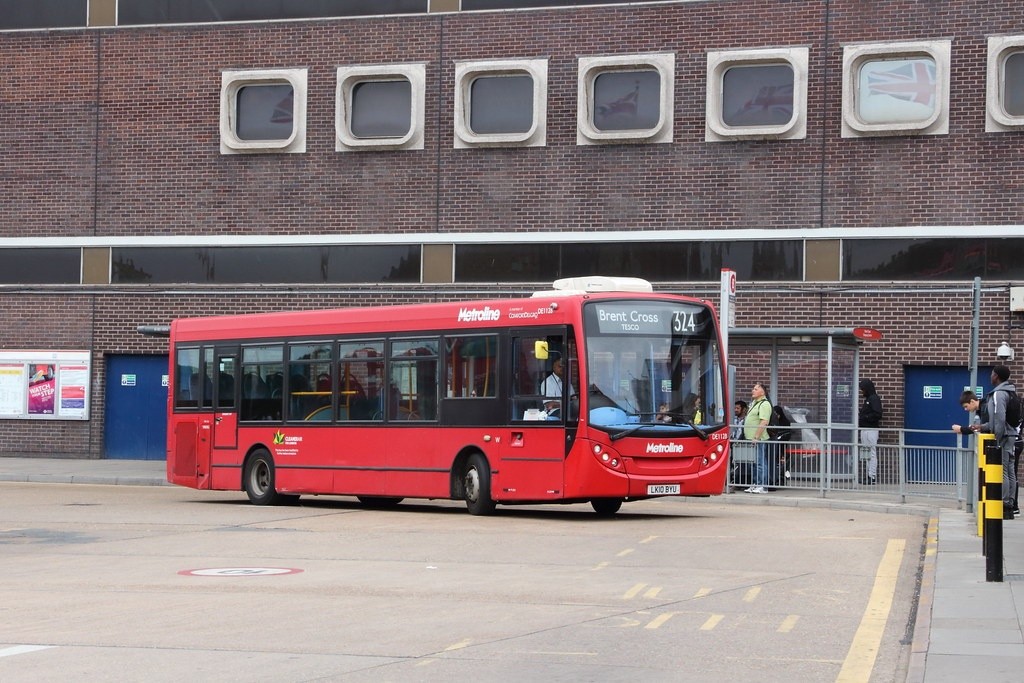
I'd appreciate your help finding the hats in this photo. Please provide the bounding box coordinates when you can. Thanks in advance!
[994,365,1010,379]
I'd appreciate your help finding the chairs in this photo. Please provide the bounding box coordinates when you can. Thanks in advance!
[189,372,365,419]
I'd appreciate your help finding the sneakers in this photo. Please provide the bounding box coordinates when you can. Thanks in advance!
[744,484,756,492]
[752,487,768,493]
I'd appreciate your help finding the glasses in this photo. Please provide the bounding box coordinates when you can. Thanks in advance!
[470,394,475,397]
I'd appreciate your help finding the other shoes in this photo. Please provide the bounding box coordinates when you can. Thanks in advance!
[769,488,776,490]
[1013,508,1020,514]
[785,471,790,478]
[859,476,875,484]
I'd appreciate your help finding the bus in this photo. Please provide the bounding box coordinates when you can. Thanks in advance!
[136,276,731,516]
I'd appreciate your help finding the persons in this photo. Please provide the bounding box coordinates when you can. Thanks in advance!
[656,382,791,494]
[952,365,1024,520]
[859,378,882,485]
[541,360,577,419]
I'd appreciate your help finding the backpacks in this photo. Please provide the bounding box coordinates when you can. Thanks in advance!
[992,389,1020,427]
[758,400,780,436]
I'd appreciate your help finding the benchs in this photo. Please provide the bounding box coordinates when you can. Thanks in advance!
[786,449,858,488]
